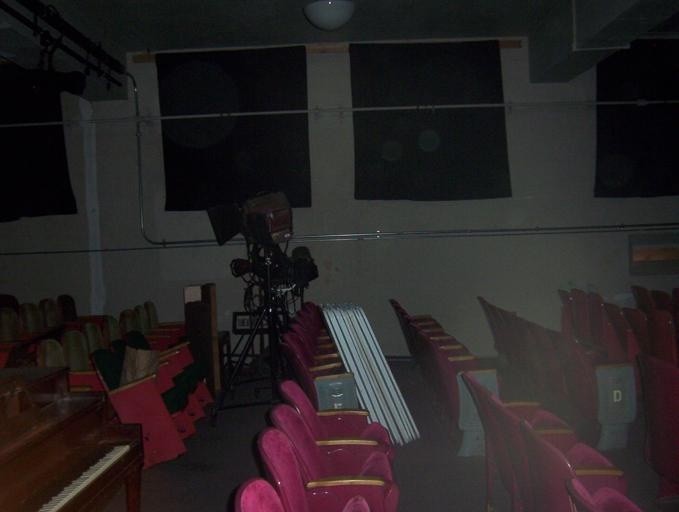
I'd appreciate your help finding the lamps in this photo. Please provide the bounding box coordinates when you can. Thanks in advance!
[301,0,356,32]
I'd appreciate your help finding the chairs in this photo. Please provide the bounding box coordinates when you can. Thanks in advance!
[461,371,541,510]
[259,427,400,511]
[478,385,578,508]
[636,351,678,503]
[233,474,370,511]
[564,476,640,509]
[268,404,395,463]
[2,292,216,472]
[277,380,394,447]
[281,286,677,440]
[522,418,628,509]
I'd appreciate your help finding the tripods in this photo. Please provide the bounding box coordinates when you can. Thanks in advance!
[208,249,280,416]
